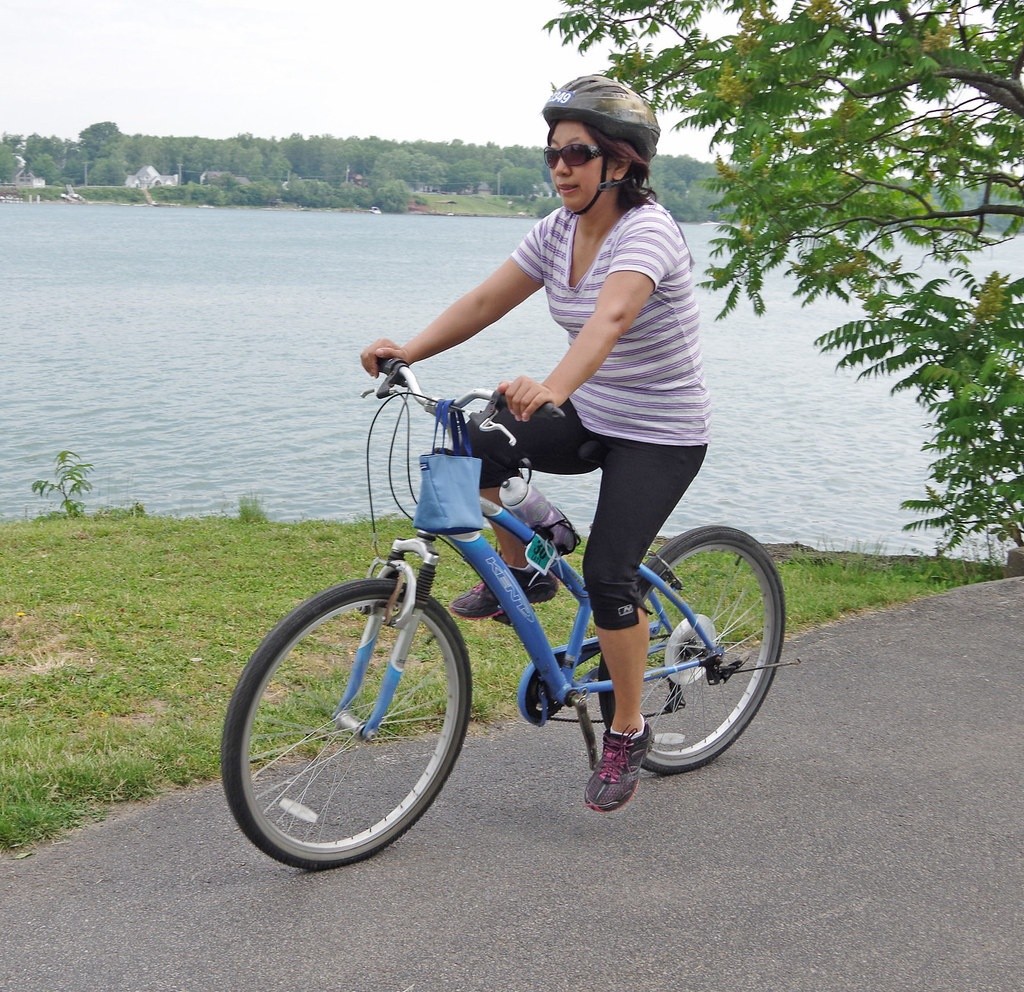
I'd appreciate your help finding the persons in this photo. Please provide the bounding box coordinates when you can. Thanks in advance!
[360,76,712,813]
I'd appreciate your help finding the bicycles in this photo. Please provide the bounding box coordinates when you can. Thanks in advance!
[218,350,790,872]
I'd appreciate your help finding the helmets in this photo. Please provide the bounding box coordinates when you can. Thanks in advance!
[542,75,662,161]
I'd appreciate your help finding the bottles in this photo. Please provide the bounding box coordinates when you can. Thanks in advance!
[499,477,578,550]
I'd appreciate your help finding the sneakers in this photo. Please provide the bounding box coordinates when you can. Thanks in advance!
[584,711,655,811]
[449,550,558,621]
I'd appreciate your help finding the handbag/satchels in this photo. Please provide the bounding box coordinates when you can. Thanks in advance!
[413,398,484,535]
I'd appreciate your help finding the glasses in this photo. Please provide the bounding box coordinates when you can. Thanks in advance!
[543,143,604,170]
